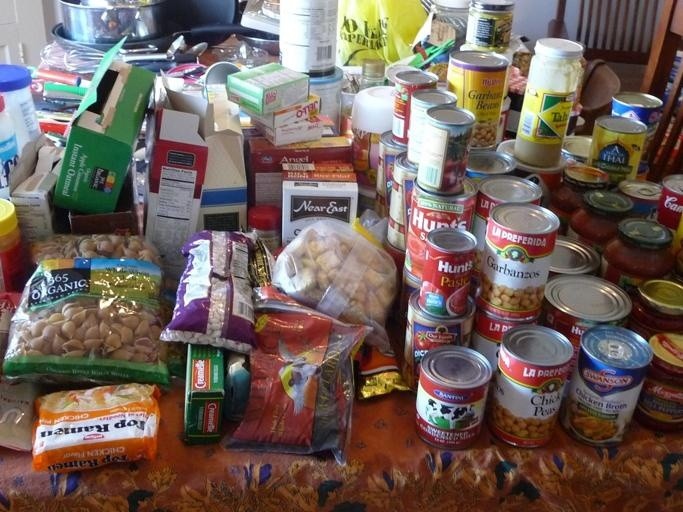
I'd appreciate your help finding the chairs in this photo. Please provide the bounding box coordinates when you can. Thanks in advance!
[639,0,683,183]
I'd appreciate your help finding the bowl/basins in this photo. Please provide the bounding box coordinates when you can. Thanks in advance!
[57,0,179,41]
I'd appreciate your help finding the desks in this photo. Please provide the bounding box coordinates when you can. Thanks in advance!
[0,319,682,512]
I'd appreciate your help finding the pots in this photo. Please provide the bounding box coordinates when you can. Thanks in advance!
[46,20,270,53]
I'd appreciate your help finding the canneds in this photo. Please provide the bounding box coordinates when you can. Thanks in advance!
[391,69,439,147]
[376,129,479,290]
[415,346,492,450]
[418,227,478,319]
[279,0,343,137]
[611,92,664,142]
[560,136,592,165]
[407,90,457,171]
[584,115,648,183]
[401,288,477,392]
[445,50,508,150]
[464,139,576,280]
[469,308,541,383]
[471,202,560,322]
[559,325,653,448]
[538,274,633,400]
[486,325,574,448]
[417,105,476,197]
[546,165,683,434]
